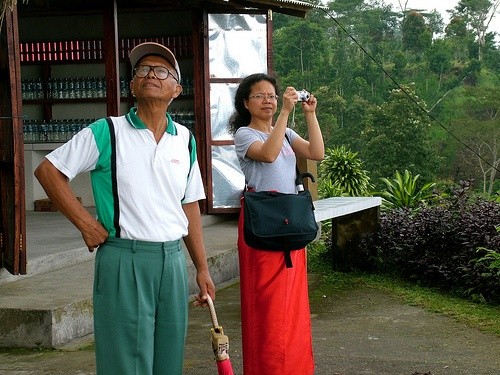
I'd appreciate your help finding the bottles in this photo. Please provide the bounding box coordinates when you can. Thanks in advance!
[121,77,134,97]
[168,108,194,130]
[21,77,106,98]
[181,72,193,93]
[22,118,93,142]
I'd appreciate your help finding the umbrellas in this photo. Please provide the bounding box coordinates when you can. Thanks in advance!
[205,294,234,375]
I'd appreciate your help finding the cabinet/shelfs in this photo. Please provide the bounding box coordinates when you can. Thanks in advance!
[117,37,195,133]
[20,41,48,142]
[47,41,112,143]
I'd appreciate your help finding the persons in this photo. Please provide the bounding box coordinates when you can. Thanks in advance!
[34,43,216,375]
[228,73,324,375]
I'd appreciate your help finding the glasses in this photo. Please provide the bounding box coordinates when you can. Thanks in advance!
[247,94,279,101]
[132,65,179,84]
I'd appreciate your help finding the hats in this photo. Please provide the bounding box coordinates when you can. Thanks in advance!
[128,42,181,105]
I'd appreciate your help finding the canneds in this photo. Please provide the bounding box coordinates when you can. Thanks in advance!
[19,40,103,62]
[119,34,192,59]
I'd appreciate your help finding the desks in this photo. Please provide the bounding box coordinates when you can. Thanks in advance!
[313,196,382,273]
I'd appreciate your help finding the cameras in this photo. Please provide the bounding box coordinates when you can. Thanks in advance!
[294,91,310,101]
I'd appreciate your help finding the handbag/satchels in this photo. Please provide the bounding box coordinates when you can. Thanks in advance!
[243,190,319,251]
